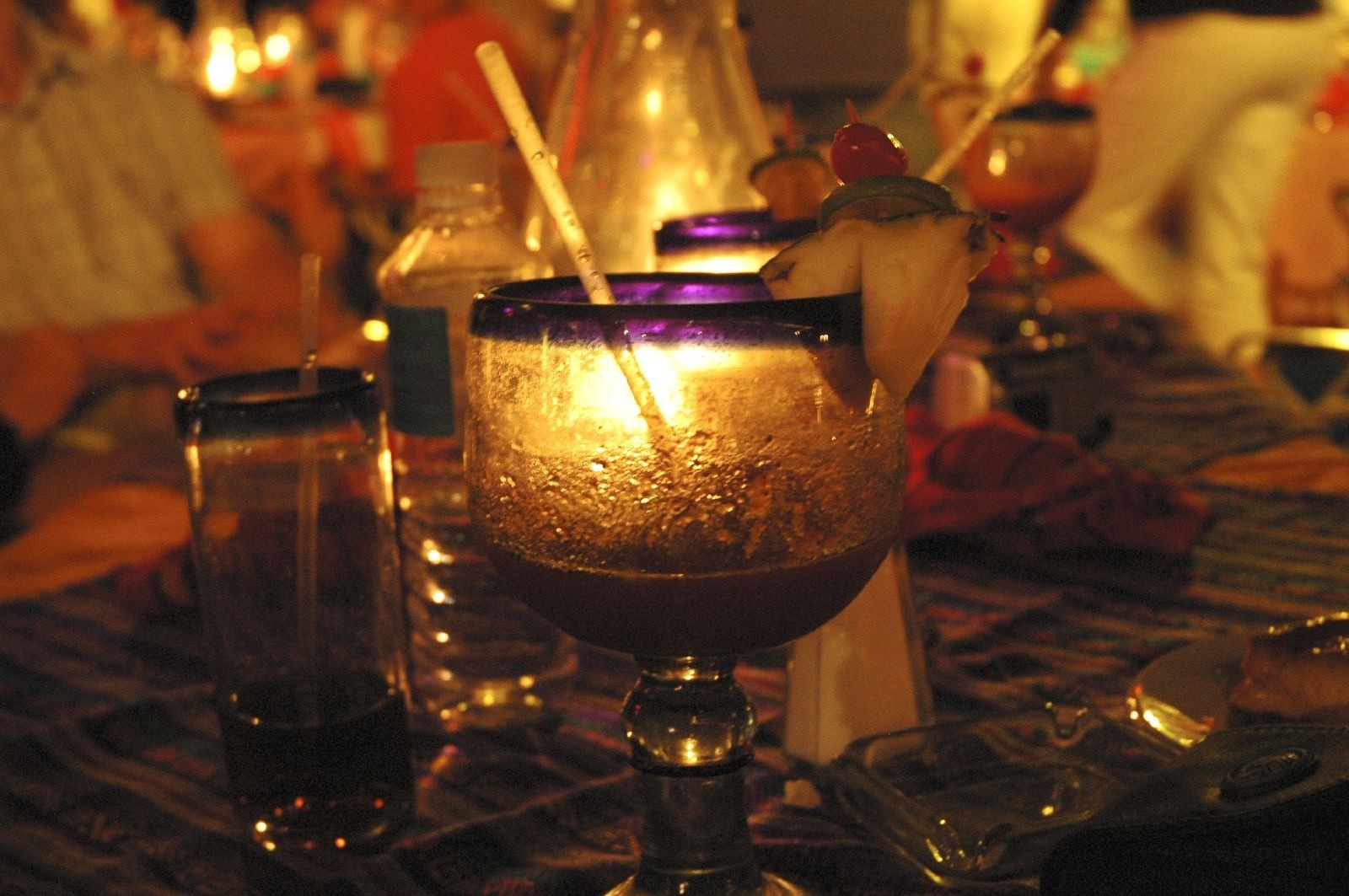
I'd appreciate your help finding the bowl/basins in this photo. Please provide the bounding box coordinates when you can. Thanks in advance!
[961,305,1163,449]
[1266,326,1349,444]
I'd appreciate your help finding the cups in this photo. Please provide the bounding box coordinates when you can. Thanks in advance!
[657,208,822,274]
[173,361,417,853]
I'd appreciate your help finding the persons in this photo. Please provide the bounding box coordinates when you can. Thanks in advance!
[317,0,553,277]
[0,0,306,528]
[0,414,43,542]
[1071,1,1349,425]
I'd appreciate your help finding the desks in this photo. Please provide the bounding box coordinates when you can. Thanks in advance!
[0,306,1349,896]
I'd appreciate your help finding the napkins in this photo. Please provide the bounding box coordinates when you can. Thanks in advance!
[907,414,1218,558]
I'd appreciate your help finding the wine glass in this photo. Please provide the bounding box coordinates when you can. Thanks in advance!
[459,274,907,896]
[954,95,1102,313]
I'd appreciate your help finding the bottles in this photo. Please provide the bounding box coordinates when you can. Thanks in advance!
[524,0,799,273]
[371,139,578,729]
